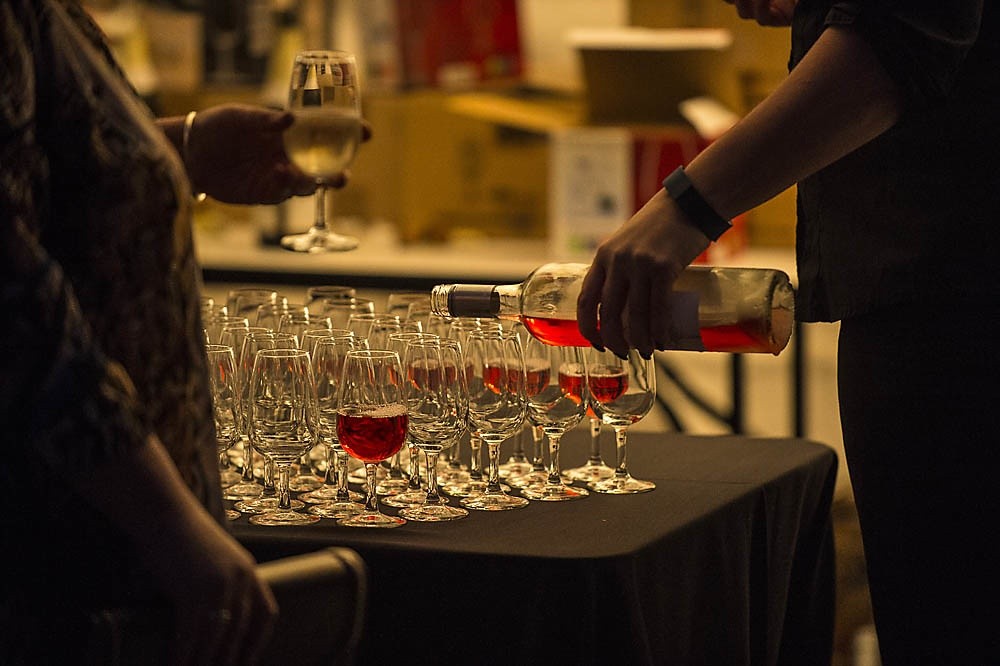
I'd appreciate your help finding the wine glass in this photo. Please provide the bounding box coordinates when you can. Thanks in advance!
[218,327,322,526]
[366,320,660,522]
[202,344,241,522]
[300,330,407,528]
[279,50,361,254]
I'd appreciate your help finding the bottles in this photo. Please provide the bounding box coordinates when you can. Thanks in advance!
[430,261,796,354]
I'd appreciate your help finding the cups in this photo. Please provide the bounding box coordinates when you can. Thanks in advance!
[202,285,500,327]
[216,316,249,389]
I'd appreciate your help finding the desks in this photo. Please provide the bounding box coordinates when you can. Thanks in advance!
[437,89,749,269]
[227,430,838,666]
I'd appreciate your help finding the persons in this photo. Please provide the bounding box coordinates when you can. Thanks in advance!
[0,0,375,666]
[576,0,1000,666]
[178,110,206,203]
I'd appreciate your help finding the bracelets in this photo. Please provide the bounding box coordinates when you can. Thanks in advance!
[662,165,733,242]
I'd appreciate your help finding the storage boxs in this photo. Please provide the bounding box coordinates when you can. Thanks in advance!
[569,25,735,121]
